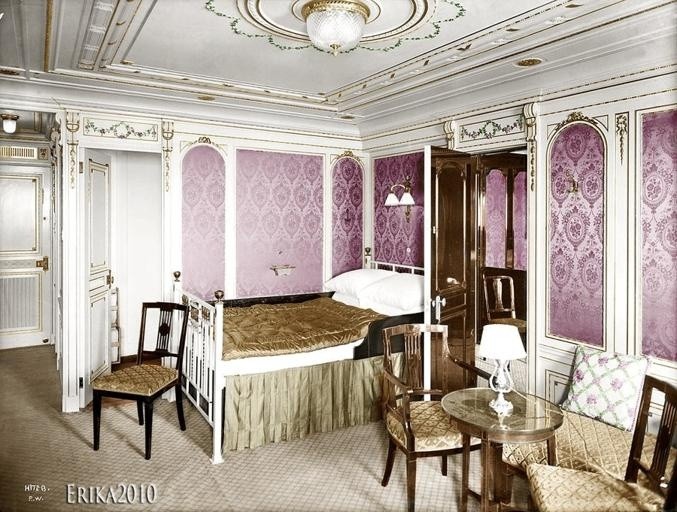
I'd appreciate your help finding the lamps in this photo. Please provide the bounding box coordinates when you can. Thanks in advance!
[301,0,370,56]
[478,324,528,407]
[2,114,19,135]
[384,182,415,206]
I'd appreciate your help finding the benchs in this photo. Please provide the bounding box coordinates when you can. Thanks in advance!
[499,410,677,491]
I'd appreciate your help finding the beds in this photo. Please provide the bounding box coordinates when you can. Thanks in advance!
[168,247,425,465]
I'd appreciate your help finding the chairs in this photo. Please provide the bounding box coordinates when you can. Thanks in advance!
[378,324,481,512]
[525,373,677,512]
[91,302,189,460]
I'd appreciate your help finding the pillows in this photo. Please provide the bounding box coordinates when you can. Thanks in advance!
[560,344,648,431]
[325,268,424,317]
